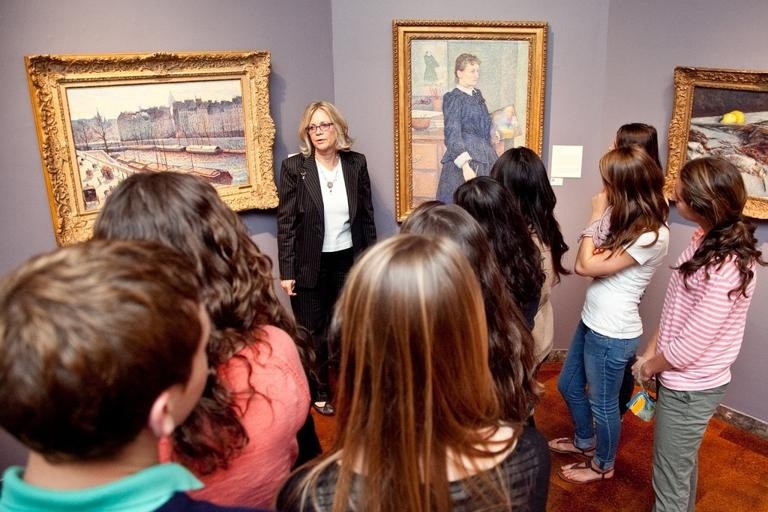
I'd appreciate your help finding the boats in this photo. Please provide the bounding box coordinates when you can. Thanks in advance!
[88,144,246,182]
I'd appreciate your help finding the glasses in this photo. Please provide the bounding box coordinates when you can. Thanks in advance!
[305,121,332,135]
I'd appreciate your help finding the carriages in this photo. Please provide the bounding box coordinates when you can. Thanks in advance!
[82,182,100,208]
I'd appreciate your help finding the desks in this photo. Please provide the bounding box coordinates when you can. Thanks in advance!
[411,119,514,198]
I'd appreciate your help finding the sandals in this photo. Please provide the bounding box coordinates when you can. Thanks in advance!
[548,436,596,457]
[558,457,614,483]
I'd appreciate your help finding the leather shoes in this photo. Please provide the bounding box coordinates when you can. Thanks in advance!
[314,402,334,414]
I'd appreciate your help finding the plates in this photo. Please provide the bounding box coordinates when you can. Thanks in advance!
[432,120,444,128]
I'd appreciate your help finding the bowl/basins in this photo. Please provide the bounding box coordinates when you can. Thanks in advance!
[412,118,430,129]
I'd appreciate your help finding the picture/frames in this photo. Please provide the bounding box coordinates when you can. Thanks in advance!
[662,65,768,221]
[23,48,280,249]
[390,17,547,224]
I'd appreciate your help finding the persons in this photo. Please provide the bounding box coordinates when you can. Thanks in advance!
[275,231,550,511]
[611,122,664,428]
[423,49,440,82]
[0,237,270,511]
[547,147,671,484]
[490,105,524,154]
[496,145,573,379]
[276,101,378,415]
[397,200,535,429]
[626,154,768,512]
[435,53,498,203]
[455,175,547,331]
[90,168,326,510]
